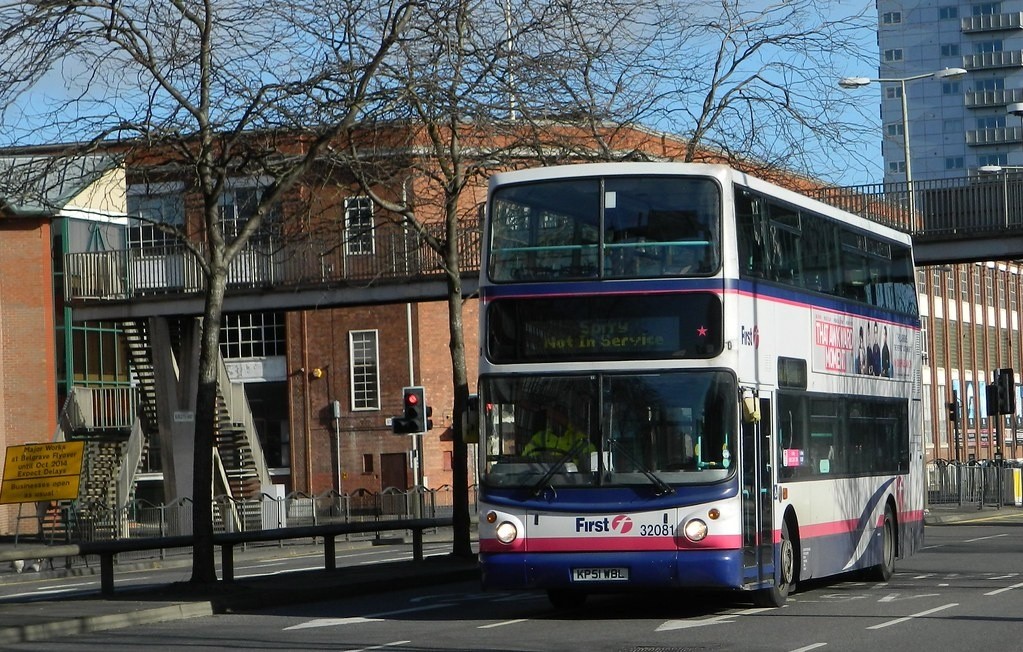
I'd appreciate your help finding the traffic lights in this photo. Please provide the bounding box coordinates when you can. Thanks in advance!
[425,405,433,430]
[391,387,427,435]
[949,403,956,422]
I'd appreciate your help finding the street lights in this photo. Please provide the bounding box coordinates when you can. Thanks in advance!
[839,67,968,234]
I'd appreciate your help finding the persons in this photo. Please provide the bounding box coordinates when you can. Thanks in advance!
[858,320,890,377]
[521,404,597,473]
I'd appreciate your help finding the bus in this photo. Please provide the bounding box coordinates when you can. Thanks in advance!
[462,161,926,607]
